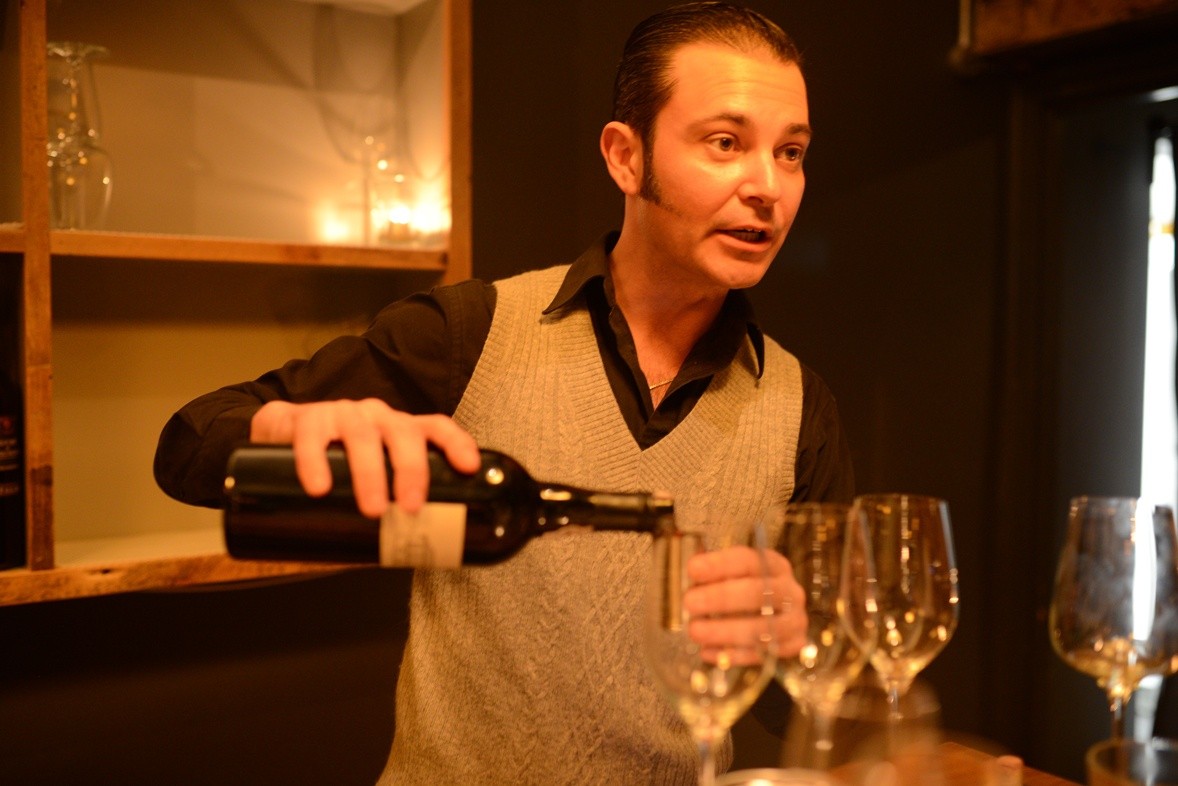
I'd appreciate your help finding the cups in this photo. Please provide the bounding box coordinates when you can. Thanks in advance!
[1085,736,1178,786]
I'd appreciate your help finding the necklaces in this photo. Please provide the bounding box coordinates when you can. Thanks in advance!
[649,377,676,391]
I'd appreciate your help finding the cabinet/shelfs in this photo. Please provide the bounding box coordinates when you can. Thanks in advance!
[0,1,472,605]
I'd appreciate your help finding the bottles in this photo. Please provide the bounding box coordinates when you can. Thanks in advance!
[222,443,677,569]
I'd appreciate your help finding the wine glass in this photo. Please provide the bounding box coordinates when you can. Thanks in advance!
[640,509,780,786]
[753,501,878,780]
[46,41,114,231]
[1047,494,1178,739]
[839,491,958,761]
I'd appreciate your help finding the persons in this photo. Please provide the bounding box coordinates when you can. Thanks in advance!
[152,9,859,786]
